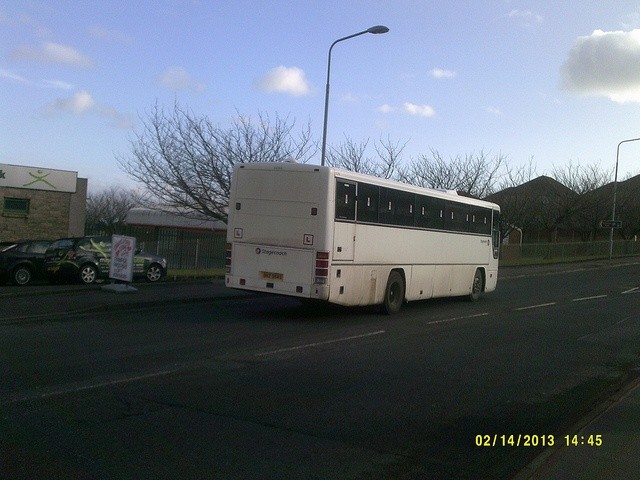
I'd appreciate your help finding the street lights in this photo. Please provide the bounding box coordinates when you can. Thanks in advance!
[596,136,639,262]
[320,25,390,167]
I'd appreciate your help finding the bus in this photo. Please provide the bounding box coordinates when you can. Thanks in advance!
[224,162,501,316]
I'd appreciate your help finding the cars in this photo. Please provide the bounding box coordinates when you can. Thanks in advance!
[0,239,51,286]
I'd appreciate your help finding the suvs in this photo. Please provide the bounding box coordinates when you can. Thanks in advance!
[38,236,168,286]
